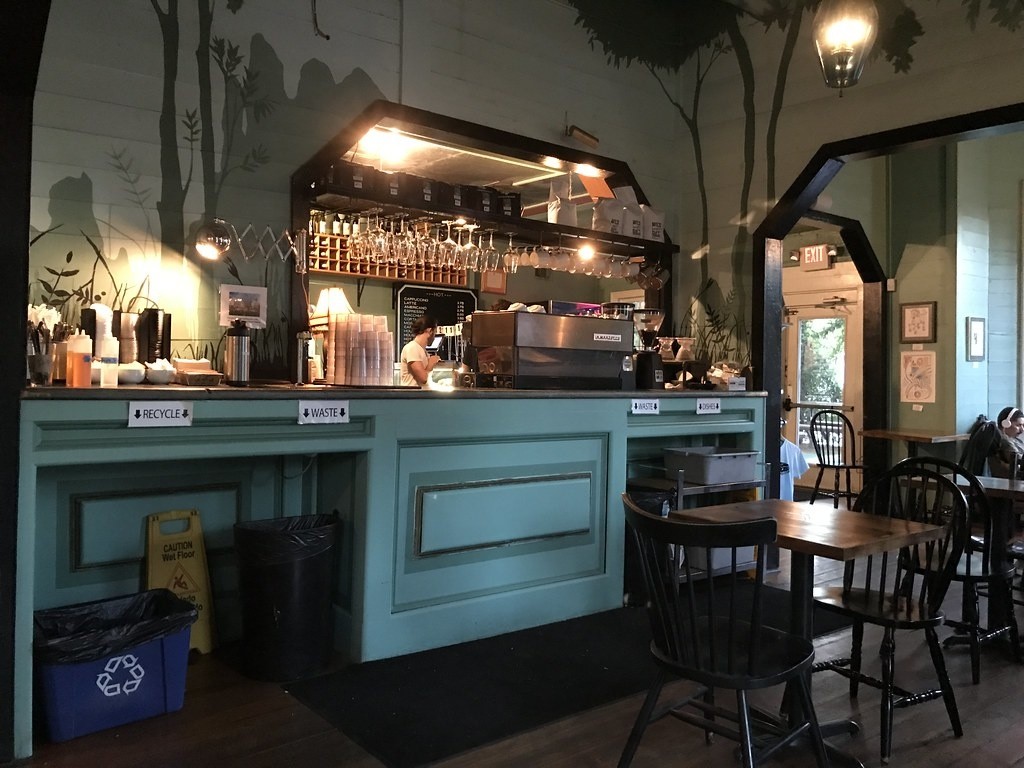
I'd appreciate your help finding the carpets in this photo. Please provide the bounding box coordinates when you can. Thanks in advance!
[280,574,865,768]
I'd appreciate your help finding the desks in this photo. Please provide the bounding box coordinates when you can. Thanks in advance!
[858,429,972,520]
[896,474,1024,663]
[667,500,947,767]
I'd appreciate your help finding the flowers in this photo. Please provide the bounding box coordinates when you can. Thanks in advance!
[27,302,60,354]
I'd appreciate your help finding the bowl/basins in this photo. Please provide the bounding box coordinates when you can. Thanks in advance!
[91,369,101,382]
[118,369,145,386]
[145,369,176,384]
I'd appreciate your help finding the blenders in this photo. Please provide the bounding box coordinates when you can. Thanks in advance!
[632,308,667,390]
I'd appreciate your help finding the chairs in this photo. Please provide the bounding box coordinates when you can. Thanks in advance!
[811,469,971,767]
[811,409,869,511]
[617,490,833,767]
[898,417,1024,645]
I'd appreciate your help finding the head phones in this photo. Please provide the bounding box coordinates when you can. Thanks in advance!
[1002,408,1019,428]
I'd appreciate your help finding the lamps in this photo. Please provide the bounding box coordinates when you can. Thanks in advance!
[195,220,233,263]
[567,125,598,149]
[813,0,880,98]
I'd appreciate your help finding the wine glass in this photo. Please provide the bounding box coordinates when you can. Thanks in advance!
[348,207,520,274]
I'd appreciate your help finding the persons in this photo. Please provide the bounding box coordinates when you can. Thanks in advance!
[460,300,514,388]
[988,407,1024,483]
[400,314,441,389]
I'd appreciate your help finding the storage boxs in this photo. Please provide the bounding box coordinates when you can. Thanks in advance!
[710,375,747,392]
[686,545,754,571]
[662,445,762,484]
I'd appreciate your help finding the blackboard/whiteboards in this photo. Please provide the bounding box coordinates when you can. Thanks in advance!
[394,282,479,363]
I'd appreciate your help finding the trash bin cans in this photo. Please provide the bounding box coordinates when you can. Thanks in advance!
[33,588,199,747]
[233,513,340,682]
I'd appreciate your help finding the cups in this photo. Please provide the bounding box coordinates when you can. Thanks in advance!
[328,313,394,386]
[505,246,670,291]
[27,354,56,388]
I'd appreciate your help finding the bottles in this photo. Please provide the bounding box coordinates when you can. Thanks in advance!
[66,327,79,386]
[101,331,119,388]
[225,318,250,386]
[308,210,449,277]
[73,330,92,387]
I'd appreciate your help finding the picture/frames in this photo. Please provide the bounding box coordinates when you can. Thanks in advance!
[899,300,938,344]
[219,283,269,331]
[965,317,986,363]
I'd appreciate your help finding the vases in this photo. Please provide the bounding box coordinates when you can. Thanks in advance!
[28,353,56,388]
